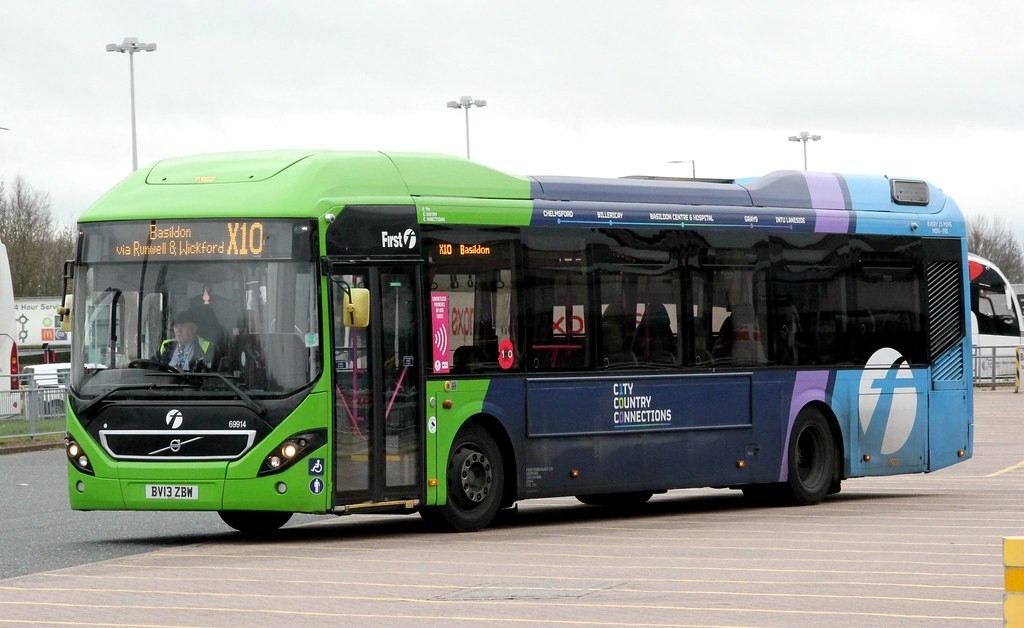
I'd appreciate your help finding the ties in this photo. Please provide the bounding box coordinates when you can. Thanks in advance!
[175,345,185,368]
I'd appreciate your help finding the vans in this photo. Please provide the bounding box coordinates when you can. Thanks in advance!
[21,362,108,412]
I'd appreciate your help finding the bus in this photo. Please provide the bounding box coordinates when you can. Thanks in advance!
[56,172,973,531]
[966,251,1024,387]
[0,244,22,418]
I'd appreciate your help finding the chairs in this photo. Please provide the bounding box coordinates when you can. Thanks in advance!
[160,301,922,414]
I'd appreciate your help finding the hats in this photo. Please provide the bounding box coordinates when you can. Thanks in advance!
[173,310,197,325]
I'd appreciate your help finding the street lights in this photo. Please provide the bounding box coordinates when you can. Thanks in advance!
[107,38,157,170]
[448,96,486,159]
[789,132,820,171]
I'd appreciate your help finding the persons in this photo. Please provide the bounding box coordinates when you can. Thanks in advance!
[142,311,219,391]
